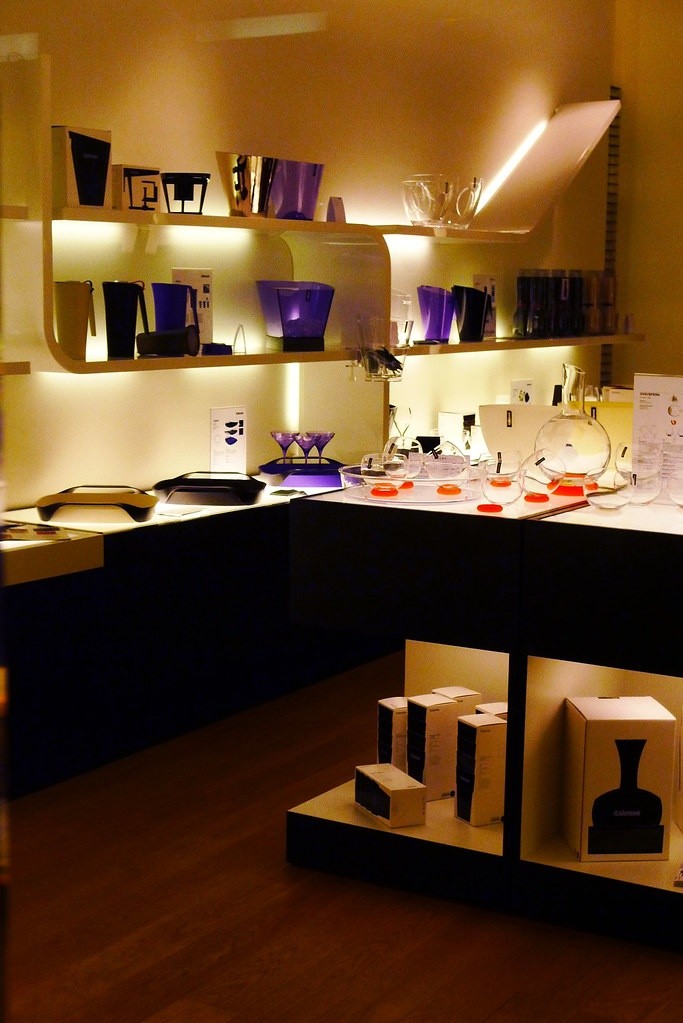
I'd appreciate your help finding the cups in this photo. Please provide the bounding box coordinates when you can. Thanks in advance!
[614,471,663,507]
[423,441,470,490]
[667,471,683,510]
[361,453,405,492]
[383,437,422,481]
[582,468,635,512]
[480,451,522,488]
[616,440,665,480]
[521,449,567,495]
[480,459,525,505]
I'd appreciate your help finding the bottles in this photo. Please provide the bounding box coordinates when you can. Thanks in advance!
[533,365,611,481]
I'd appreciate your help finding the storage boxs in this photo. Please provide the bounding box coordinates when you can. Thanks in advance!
[629,372,682,506]
[564,694,676,862]
[354,762,427,828]
[602,385,633,404]
[406,694,463,801]
[378,696,408,776]
[431,686,482,717]
[474,702,508,724]
[453,712,508,827]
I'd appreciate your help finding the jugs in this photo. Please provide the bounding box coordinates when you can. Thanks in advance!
[52,281,199,360]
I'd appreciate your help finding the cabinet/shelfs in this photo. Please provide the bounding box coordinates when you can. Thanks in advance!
[282,458,682,953]
[0,31,625,802]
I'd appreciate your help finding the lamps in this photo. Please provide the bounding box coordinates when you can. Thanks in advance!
[468,121,547,216]
[282,362,301,464]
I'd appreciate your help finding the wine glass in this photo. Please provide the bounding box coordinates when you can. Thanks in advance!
[306,433,335,463]
[270,431,300,463]
[293,436,322,463]
